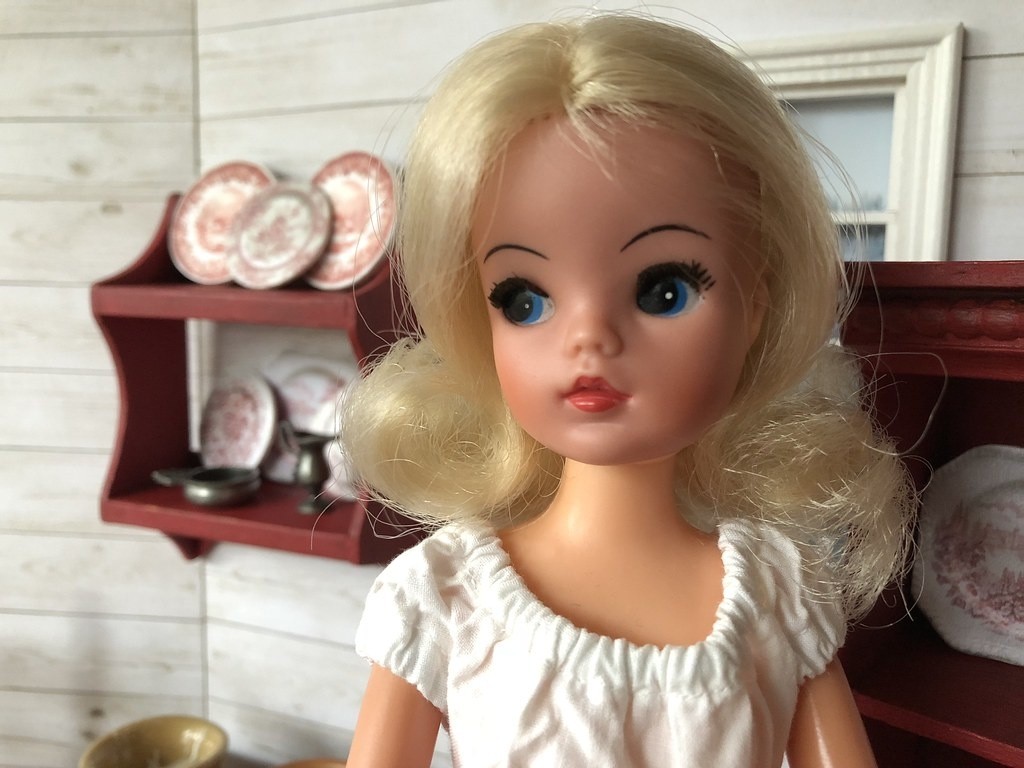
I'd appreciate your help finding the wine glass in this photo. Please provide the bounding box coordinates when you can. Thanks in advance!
[293,431,338,514]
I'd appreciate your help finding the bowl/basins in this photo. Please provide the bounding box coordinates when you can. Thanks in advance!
[74,714,229,767]
[179,464,261,508]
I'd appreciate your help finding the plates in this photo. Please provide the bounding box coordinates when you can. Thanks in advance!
[910,444,1024,669]
[267,355,359,434]
[200,366,279,469]
[165,161,277,286]
[301,151,397,292]
[224,179,332,290]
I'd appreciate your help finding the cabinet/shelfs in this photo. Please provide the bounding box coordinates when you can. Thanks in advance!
[91,191,433,566]
[822,259,1024,768]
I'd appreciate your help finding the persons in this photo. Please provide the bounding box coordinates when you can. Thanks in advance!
[311,3,948,768]
